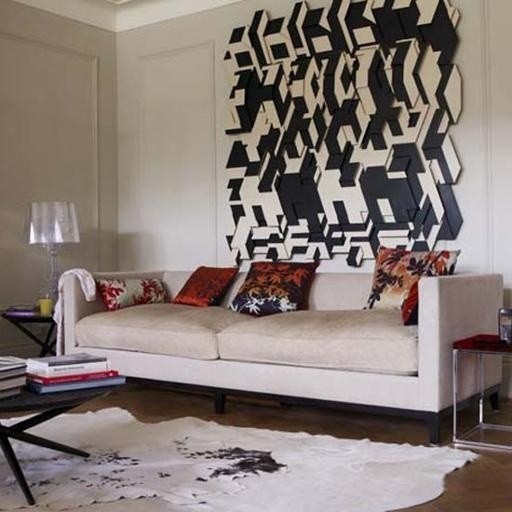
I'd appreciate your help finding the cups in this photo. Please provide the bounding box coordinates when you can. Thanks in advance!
[40,300,52,318]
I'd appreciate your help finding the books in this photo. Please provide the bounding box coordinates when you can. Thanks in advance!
[0,351,126,398]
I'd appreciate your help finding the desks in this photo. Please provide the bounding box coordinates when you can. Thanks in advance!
[0,308,57,358]
[451,333,512,452]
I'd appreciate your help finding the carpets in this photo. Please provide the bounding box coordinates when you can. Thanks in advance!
[1,406,479,511]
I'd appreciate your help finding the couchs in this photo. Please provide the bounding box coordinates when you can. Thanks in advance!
[62,270,504,446]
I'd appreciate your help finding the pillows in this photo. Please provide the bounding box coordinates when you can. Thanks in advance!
[94,261,319,318]
[362,243,461,327]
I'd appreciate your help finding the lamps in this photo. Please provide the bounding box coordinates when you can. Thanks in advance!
[24,200,81,310]
